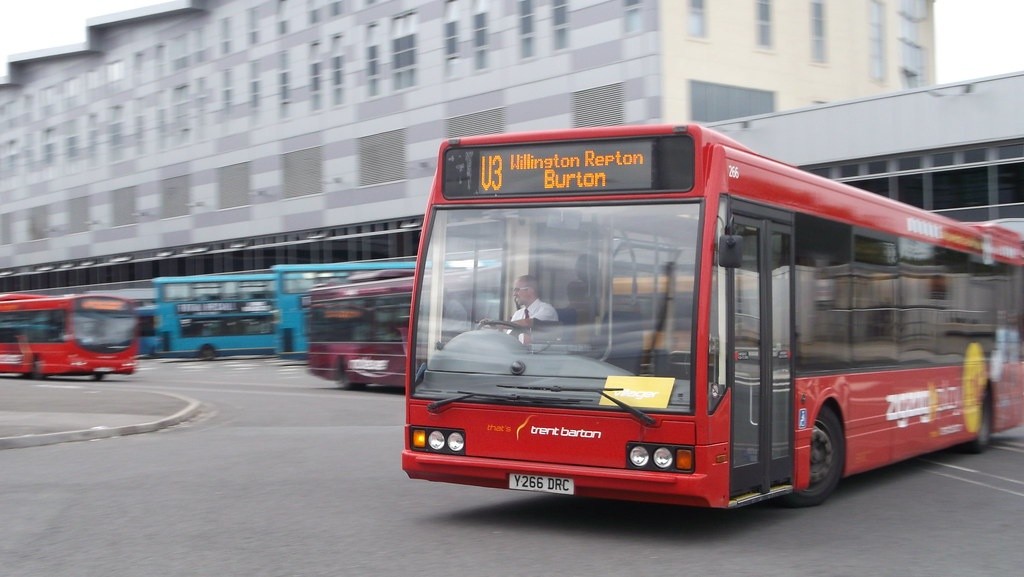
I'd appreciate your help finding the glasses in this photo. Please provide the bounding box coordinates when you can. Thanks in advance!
[512,287,528,293]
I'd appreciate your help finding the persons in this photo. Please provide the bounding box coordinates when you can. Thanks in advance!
[568,256,600,356]
[478,275,561,351]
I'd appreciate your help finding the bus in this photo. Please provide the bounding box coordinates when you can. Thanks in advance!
[300,266,416,392]
[151,272,287,362]
[270,260,419,360]
[0,292,140,381]
[401,124,1024,509]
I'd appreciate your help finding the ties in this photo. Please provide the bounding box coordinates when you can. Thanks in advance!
[522,309,531,345]
